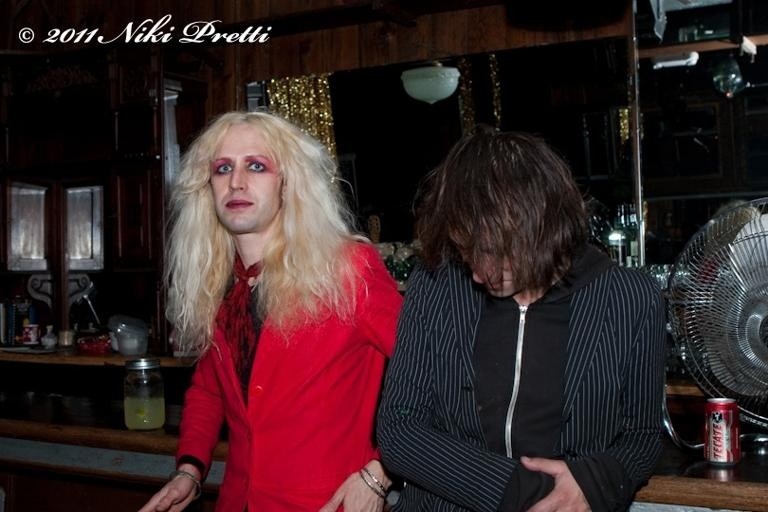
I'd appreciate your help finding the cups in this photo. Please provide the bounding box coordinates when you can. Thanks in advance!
[22,324,42,346]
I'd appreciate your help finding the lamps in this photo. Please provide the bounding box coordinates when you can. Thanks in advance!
[400,59,460,106]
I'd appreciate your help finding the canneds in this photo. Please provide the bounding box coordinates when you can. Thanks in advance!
[705,398,742,466]
[601,233,627,268]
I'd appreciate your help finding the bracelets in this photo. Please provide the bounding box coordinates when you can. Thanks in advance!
[362,466,388,496]
[169,470,202,501]
[358,470,385,500]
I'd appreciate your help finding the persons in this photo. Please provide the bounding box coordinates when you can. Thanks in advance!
[140,108,405,512]
[376,123,671,510]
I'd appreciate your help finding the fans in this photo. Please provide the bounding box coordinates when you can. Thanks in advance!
[664,197,767,459]
